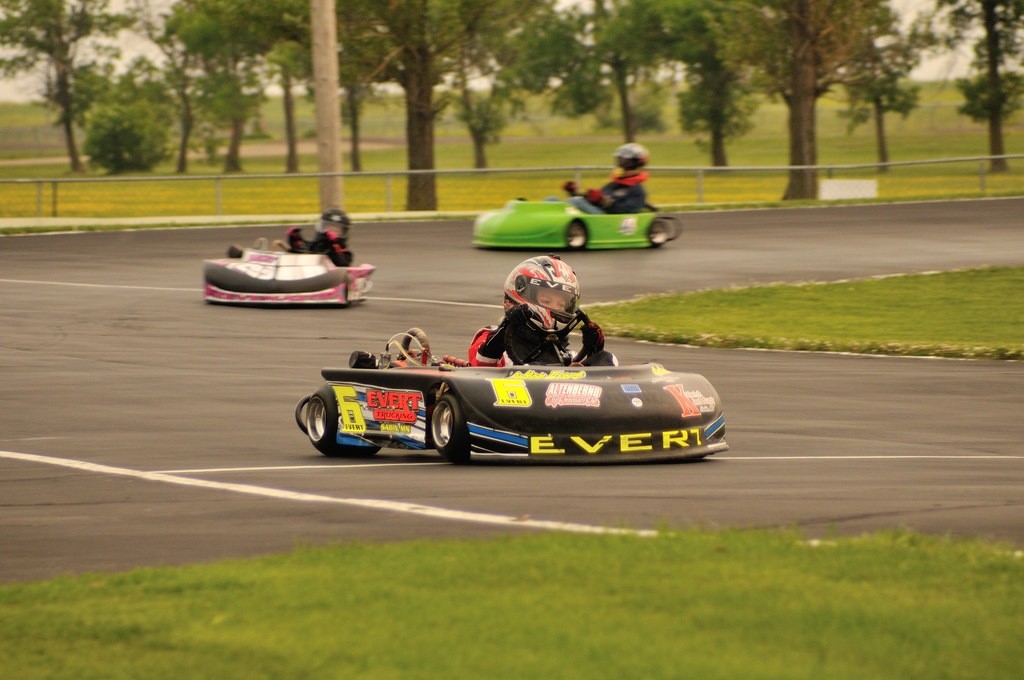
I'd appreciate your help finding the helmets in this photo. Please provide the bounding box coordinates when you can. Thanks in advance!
[503,254,582,346]
[612,143,650,178]
[315,206,351,244]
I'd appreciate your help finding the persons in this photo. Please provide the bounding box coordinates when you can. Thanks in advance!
[286,208,353,267]
[467,253,619,367]
[561,143,651,216]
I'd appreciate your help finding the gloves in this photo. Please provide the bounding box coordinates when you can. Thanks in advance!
[287,227,302,245]
[321,231,338,246]
[581,322,605,354]
[586,189,603,204]
[475,303,533,364]
[561,179,577,193]
